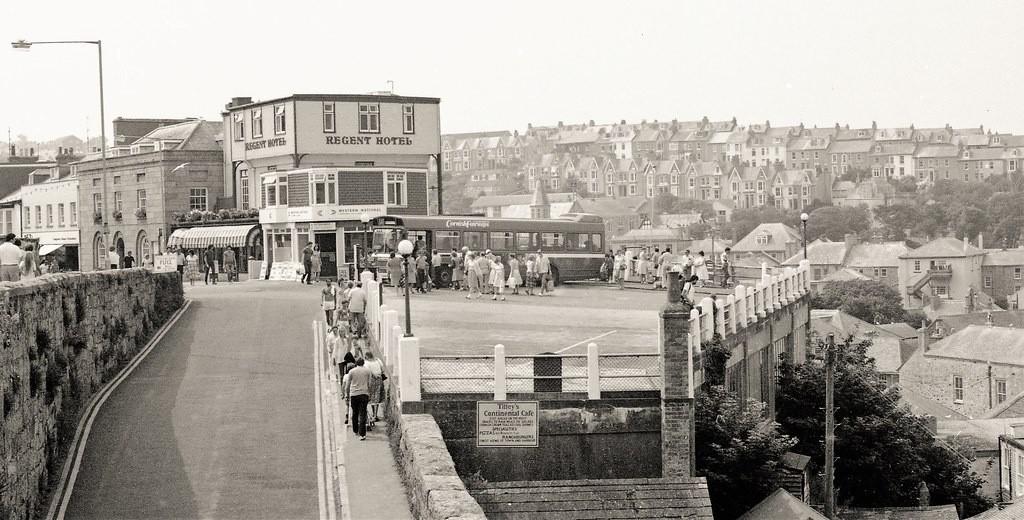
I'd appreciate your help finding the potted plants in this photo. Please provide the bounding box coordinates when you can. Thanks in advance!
[92,211,102,222]
[112,211,122,221]
[134,208,146,219]
[173,208,258,223]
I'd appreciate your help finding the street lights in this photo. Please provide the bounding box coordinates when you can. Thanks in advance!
[11,40,111,270]
[706,228,720,260]
[398,239,414,338]
[361,213,371,272]
[800,213,808,259]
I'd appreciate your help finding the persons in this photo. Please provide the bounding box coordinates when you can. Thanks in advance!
[417,235,425,251]
[449,245,552,301]
[162,247,199,285]
[605,246,710,290]
[696,293,717,312]
[301,241,321,285]
[431,249,442,289]
[720,247,731,289]
[387,252,427,296]
[320,276,386,438]
[584,241,599,252]
[203,244,217,285]
[367,250,374,264]
[123,251,135,269]
[109,245,119,269]
[0,232,59,282]
[681,275,699,308]
[223,245,237,283]
[142,254,153,267]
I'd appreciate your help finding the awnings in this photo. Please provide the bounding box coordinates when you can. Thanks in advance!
[166,225,261,248]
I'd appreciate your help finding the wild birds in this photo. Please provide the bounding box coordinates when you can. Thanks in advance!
[172,162,190,172]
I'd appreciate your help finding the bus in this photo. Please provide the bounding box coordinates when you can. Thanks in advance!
[370,212,607,287]
